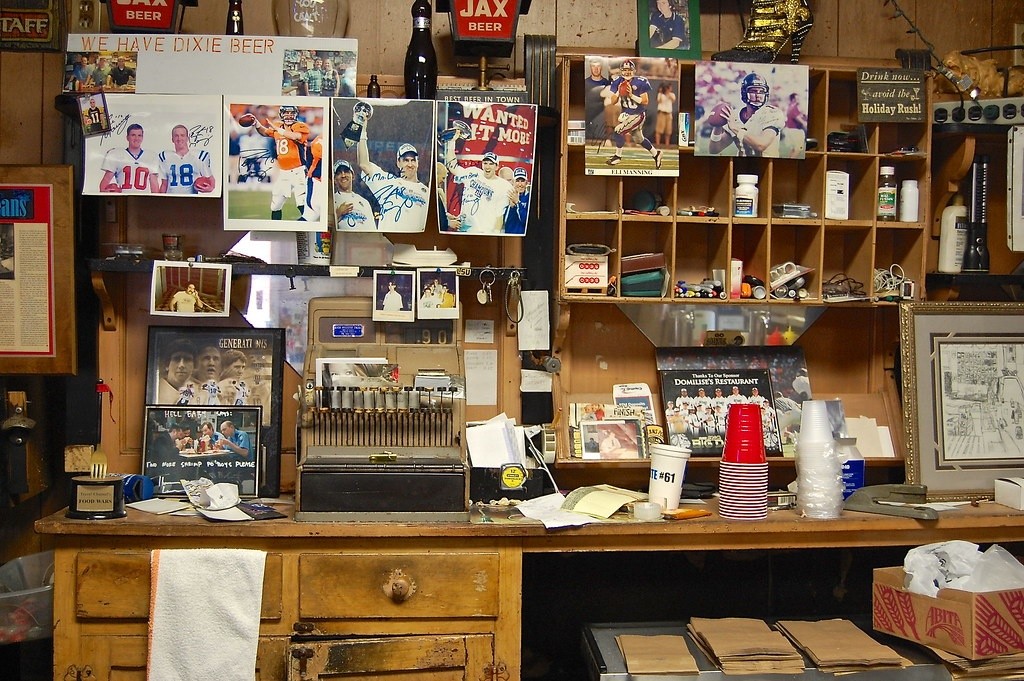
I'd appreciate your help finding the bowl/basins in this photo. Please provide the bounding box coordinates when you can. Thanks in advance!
[162,234,184,251]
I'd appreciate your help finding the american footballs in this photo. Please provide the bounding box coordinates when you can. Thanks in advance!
[707,102,732,128]
[194,177,212,191]
[618,80,631,98]
[238,114,255,128]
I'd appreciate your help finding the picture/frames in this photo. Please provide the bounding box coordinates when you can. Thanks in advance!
[636,0,702,60]
[898,301,1024,502]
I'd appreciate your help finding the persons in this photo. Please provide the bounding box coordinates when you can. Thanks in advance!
[147,412,254,489]
[435,123,529,236]
[63,50,137,93]
[98,121,216,196]
[382,279,405,312]
[249,104,323,221]
[649,0,689,51]
[695,62,808,159]
[168,281,204,314]
[587,437,600,452]
[303,58,341,98]
[420,277,456,309]
[585,55,677,171]
[659,350,798,389]
[333,117,429,233]
[774,368,812,447]
[582,402,605,421]
[665,386,779,451]
[156,336,254,406]
[86,97,103,132]
[599,430,622,456]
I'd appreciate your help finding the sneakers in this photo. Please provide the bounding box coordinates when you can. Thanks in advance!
[652,150,663,170]
[606,154,622,166]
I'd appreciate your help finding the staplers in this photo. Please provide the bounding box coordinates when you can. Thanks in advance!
[843,484,938,520]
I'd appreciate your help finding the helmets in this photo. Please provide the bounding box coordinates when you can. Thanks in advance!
[619,58,636,81]
[741,70,770,110]
[278,105,299,122]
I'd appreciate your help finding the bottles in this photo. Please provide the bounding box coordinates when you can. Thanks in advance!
[935,194,970,272]
[367,74,380,98]
[735,174,760,217]
[226,0,244,35]
[877,165,896,222]
[899,181,920,224]
[404,0,438,100]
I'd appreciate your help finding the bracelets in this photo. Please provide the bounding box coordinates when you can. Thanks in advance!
[358,131,368,140]
[710,128,722,142]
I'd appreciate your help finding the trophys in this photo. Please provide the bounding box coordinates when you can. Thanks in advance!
[434,118,472,147]
[339,102,373,150]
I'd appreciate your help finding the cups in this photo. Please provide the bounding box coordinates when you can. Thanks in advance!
[719,398,841,521]
[647,441,693,513]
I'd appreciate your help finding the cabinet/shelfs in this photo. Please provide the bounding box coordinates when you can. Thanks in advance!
[34,35,1024,681]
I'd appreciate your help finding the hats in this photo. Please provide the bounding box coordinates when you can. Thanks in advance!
[481,152,499,166]
[698,388,704,392]
[681,388,687,392]
[715,388,722,392]
[762,399,770,403]
[397,143,419,163]
[334,159,355,175]
[682,400,690,405]
[752,387,758,391]
[732,386,738,389]
[667,401,674,405]
[513,166,528,181]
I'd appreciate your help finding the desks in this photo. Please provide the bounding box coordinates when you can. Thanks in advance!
[578,617,960,681]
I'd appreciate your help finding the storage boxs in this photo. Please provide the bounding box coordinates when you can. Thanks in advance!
[565,255,608,288]
[825,171,849,220]
[0,549,55,645]
[873,566,1024,660]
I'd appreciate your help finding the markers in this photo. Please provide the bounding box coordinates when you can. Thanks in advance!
[678,209,717,217]
[675,277,728,298]
